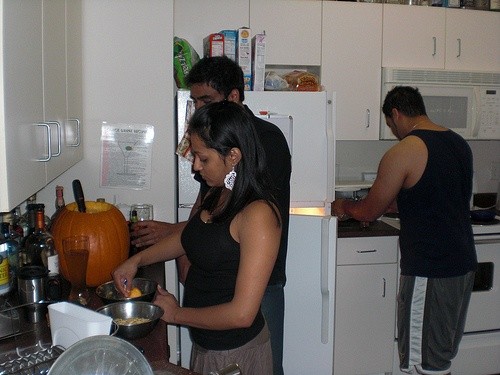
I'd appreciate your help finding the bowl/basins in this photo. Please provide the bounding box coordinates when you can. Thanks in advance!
[95,279,159,304]
[95,301,165,340]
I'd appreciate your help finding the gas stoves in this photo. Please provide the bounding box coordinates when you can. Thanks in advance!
[378,202,500,237]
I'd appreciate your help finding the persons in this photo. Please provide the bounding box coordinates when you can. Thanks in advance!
[332,86,478,375]
[129,56,291,375]
[113,101,283,375]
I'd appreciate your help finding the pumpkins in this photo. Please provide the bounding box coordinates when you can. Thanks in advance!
[52,201,130,287]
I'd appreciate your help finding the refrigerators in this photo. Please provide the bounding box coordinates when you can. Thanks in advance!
[176,88,338,374]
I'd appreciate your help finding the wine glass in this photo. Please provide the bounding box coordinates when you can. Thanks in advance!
[62,235,93,306]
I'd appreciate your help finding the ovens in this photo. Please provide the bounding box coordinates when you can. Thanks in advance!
[451,235,500,374]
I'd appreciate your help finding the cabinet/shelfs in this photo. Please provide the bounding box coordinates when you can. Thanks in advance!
[380,3,500,72]
[322,1,382,140]
[0,0,67,212]
[174,0,324,66]
[333,238,402,375]
[66,0,82,169]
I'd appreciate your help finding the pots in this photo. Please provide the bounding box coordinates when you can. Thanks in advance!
[468,209,496,221]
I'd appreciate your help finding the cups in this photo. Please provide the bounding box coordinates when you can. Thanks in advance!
[17,265,51,326]
[129,203,155,229]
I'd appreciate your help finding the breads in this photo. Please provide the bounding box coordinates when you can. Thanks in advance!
[286,71,319,91]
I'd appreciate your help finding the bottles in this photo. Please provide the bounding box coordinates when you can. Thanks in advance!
[0,185,67,301]
[96,197,107,204]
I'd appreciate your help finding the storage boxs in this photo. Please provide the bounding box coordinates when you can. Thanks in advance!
[202,26,267,92]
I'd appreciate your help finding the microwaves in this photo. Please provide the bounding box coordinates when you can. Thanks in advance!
[380,67,500,141]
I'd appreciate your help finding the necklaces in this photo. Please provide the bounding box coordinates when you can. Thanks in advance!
[408,121,423,132]
[203,213,212,223]
